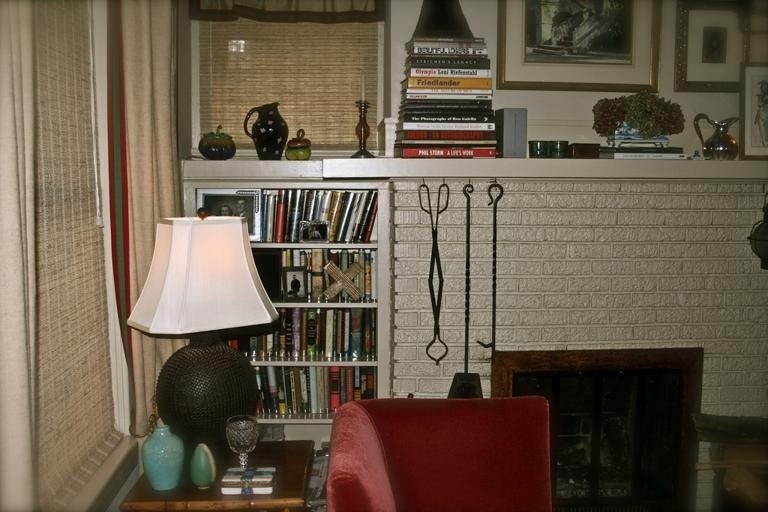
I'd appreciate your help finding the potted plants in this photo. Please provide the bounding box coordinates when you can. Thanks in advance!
[591,92,687,149]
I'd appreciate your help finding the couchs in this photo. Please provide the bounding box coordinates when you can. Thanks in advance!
[327,395,552,511]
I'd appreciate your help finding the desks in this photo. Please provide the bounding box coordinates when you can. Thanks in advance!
[119,439,314,512]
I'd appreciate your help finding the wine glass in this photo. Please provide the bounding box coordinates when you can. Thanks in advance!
[226,415,259,472]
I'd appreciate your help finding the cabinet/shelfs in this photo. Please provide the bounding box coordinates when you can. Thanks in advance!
[187,184,391,417]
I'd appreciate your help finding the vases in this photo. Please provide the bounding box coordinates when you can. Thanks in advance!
[140,424,185,493]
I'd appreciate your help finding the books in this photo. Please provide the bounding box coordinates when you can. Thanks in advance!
[280,250,377,303]
[393,36,502,159]
[599,147,688,160]
[260,189,378,243]
[253,366,377,418]
[227,307,377,361]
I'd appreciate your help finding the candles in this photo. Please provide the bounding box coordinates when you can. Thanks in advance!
[360,71,367,102]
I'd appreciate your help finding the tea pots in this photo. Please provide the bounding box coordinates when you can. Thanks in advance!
[694,112,739,160]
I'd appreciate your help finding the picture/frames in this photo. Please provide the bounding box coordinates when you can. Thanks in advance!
[281,264,309,303]
[672,1,751,95]
[298,219,331,244]
[738,61,768,162]
[192,187,262,242]
[496,0,664,90]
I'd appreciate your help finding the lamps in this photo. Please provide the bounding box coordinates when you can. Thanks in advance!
[127,206,279,469]
